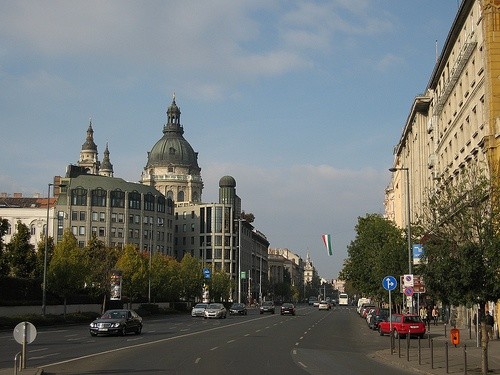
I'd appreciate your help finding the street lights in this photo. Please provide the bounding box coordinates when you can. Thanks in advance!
[41,183,67,318]
[203,242,213,302]
[234,218,246,303]
[388,167,413,314]
[148,222,163,303]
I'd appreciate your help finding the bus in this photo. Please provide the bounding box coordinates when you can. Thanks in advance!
[339,293,348,306]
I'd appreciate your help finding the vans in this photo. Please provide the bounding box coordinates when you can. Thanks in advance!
[358,298,371,315]
[309,297,316,305]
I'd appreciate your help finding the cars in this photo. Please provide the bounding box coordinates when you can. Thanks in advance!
[318,301,331,311]
[360,303,395,331]
[229,303,247,316]
[280,303,295,316]
[191,304,208,317]
[378,314,426,339]
[260,301,275,314]
[314,301,319,307]
[204,304,226,319]
[89,310,143,337]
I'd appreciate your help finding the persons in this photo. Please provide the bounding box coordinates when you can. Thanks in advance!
[432,305,440,326]
[420,306,428,326]
[474,309,481,333]
[486,311,494,341]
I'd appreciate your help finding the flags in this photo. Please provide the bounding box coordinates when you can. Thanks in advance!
[322,234,333,256]
